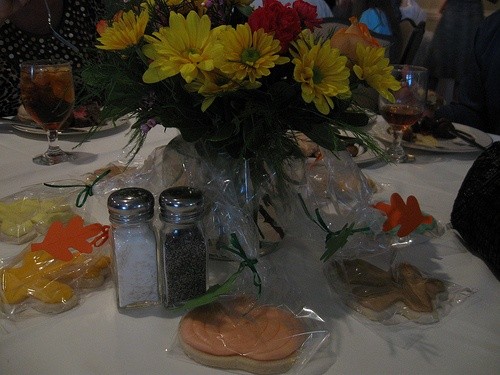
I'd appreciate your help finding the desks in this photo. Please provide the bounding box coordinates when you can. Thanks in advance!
[0,116,500,375]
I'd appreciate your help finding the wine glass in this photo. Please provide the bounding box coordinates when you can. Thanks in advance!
[20,58,77,165]
[377,65,432,162]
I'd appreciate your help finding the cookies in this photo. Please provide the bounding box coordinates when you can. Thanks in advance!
[323,258,450,324]
[0,198,111,318]
[177,293,314,374]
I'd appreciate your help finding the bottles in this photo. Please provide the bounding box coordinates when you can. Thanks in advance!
[158,187,209,309]
[107,187,161,308]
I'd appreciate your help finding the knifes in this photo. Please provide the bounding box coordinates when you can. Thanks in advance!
[1,118,87,132]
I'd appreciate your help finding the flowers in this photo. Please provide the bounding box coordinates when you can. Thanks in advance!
[71,0,445,311]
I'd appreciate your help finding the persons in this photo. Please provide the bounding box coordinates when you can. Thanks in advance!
[249,0,402,71]
[425,0,500,137]
[0,0,117,136]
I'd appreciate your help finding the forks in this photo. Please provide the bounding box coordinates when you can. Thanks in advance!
[45,0,79,53]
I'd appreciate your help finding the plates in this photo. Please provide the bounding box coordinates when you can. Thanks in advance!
[9,105,129,136]
[373,115,494,153]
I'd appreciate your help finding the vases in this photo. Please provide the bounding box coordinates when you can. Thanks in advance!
[159,133,262,246]
